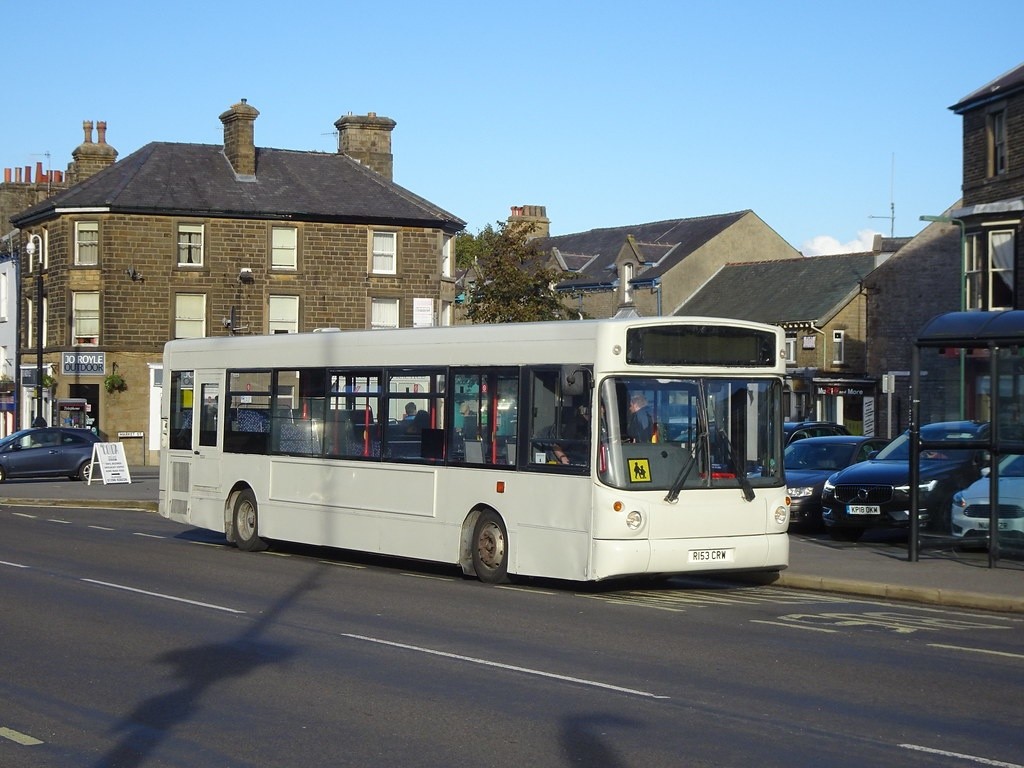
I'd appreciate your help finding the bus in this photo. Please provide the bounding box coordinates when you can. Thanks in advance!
[157,315,821,588]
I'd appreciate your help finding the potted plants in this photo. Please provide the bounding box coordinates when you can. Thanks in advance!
[104,372,125,393]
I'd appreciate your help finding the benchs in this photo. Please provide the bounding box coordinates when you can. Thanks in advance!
[182,402,506,465]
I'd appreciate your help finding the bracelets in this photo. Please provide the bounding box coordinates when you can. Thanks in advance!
[560,454,566,460]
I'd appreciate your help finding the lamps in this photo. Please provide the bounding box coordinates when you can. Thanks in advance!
[237,268,256,284]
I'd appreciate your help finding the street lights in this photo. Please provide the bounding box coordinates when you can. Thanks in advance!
[25,232,43,431]
[918,215,967,424]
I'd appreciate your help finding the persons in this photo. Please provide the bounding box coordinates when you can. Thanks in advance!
[456,403,478,440]
[553,395,609,468]
[404,402,417,420]
[626,396,654,443]
[30,436,41,448]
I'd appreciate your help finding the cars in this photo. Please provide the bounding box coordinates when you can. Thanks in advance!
[745,435,907,528]
[648,421,854,489]
[0,427,107,482]
[820,420,1024,538]
[949,453,1024,555]
